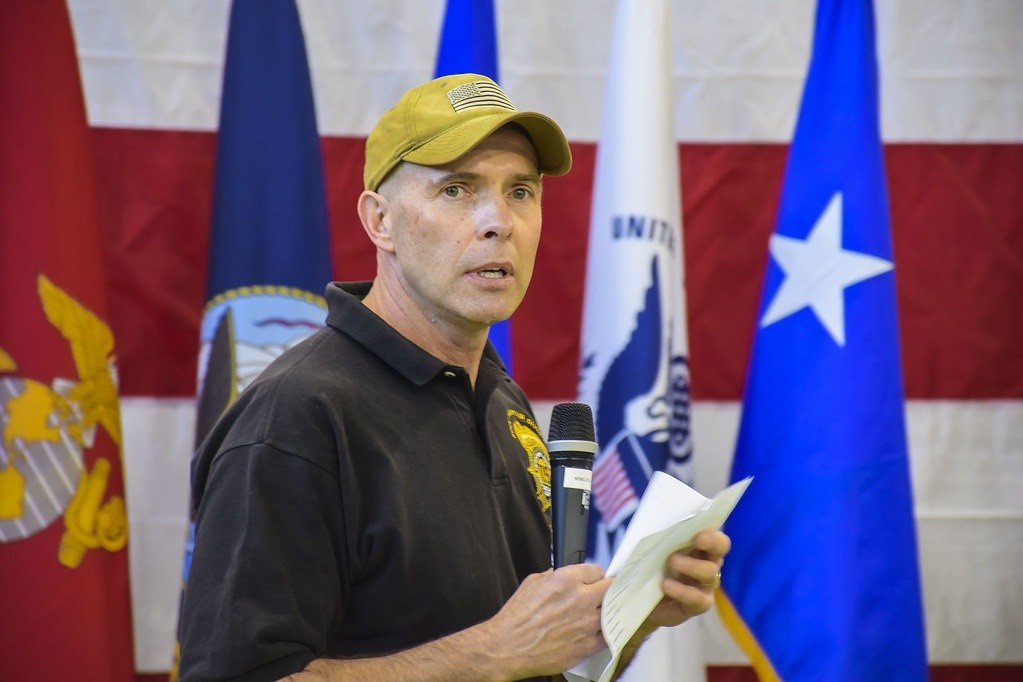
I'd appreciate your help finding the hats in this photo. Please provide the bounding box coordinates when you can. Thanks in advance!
[363,73,572,193]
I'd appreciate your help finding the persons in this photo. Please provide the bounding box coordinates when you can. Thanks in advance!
[180,71,734,682]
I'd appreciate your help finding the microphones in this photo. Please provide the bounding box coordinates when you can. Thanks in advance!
[545,402,599,569]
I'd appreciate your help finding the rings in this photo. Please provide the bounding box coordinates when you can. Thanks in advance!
[702,571,721,588]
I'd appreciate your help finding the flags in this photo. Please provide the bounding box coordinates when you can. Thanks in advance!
[574,0,706,682]
[1,0,140,682]
[713,0,927,682]
[410,0,524,376]
[169,0,335,682]
